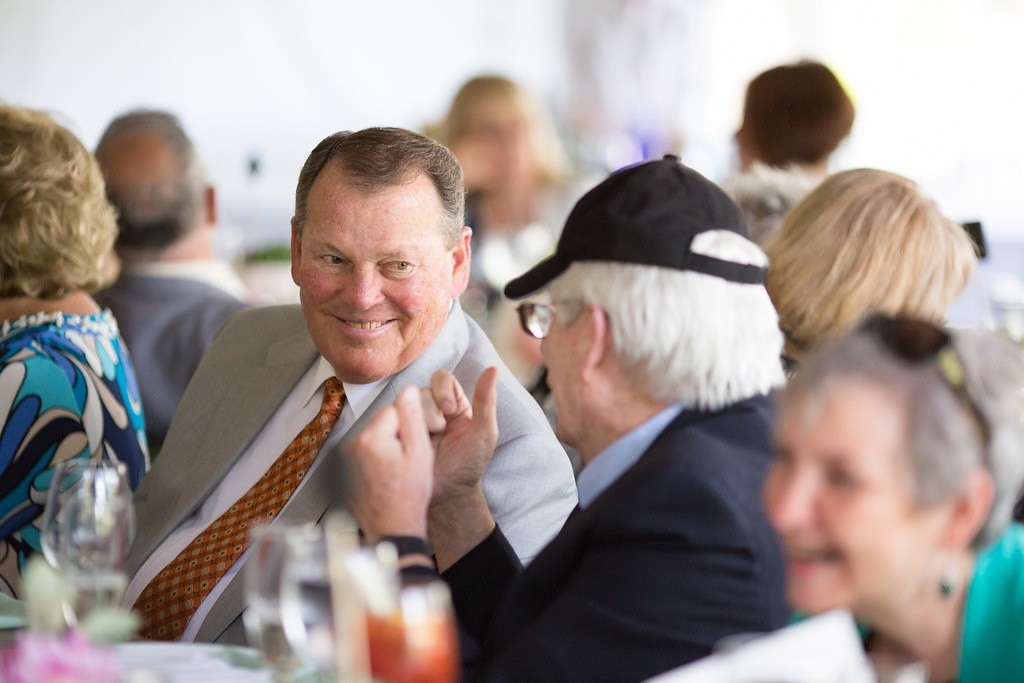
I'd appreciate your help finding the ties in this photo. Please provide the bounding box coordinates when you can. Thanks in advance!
[129,376,347,644]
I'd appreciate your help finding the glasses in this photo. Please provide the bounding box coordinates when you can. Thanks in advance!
[513,302,587,339]
[853,310,993,470]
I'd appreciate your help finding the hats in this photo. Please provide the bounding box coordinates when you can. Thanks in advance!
[504,154,769,299]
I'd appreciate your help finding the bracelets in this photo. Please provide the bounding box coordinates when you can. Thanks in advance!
[377,535,441,558]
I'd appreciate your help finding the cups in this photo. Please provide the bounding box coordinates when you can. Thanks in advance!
[357,579,461,683]
[246,519,343,683]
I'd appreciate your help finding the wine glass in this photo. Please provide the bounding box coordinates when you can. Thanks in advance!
[41,455,140,620]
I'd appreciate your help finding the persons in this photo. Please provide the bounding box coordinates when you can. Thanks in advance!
[338,151,795,683]
[713,56,854,262]
[755,304,1023,683]
[116,126,578,651]
[406,76,583,411]
[87,112,265,464]
[755,164,982,376]
[0,105,151,630]
